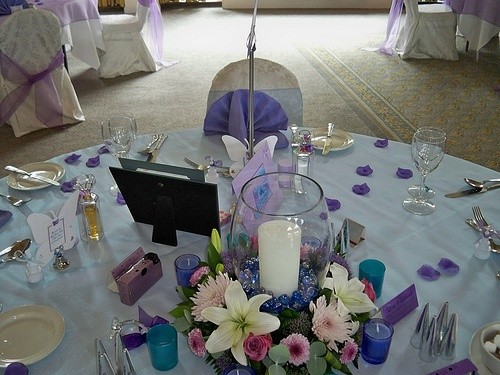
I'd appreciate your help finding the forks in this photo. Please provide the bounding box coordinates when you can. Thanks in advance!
[472,205,493,231]
[0,193,32,206]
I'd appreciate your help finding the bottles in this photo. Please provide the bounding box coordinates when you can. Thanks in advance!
[290,123,312,177]
[70,173,104,243]
[410,302,459,361]
[96,332,137,375]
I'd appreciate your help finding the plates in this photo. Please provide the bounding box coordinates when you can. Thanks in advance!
[309,126,353,151]
[468,321,500,375]
[6,162,65,190]
[0,304,66,368]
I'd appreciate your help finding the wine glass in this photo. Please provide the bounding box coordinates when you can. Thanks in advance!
[402,126,447,216]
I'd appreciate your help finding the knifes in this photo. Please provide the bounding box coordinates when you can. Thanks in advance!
[445,184,500,198]
[147,133,168,162]
[321,123,335,155]
[5,166,61,187]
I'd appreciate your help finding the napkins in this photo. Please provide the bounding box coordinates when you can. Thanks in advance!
[203,88,289,150]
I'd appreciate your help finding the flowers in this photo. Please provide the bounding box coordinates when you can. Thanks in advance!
[172,225,383,375]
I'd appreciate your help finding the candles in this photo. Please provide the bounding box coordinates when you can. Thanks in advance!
[366,322,390,359]
[257,219,301,297]
[177,258,198,286]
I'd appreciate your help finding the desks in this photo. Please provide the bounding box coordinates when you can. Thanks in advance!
[0,0,102,72]
[443,0,500,63]
[0,126,500,375]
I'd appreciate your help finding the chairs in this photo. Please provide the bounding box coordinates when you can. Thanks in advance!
[396,0,459,61]
[206,57,304,127]
[98,0,163,80]
[0,8,85,137]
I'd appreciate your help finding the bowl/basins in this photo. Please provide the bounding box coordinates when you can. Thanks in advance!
[479,324,500,375]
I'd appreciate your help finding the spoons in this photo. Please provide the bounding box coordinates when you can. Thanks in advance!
[0,238,32,264]
[464,177,500,188]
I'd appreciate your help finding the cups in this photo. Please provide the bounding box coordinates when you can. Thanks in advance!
[359,259,387,301]
[175,254,201,286]
[231,172,334,315]
[361,318,394,365]
[222,363,254,375]
[101,112,137,159]
[146,323,178,371]
